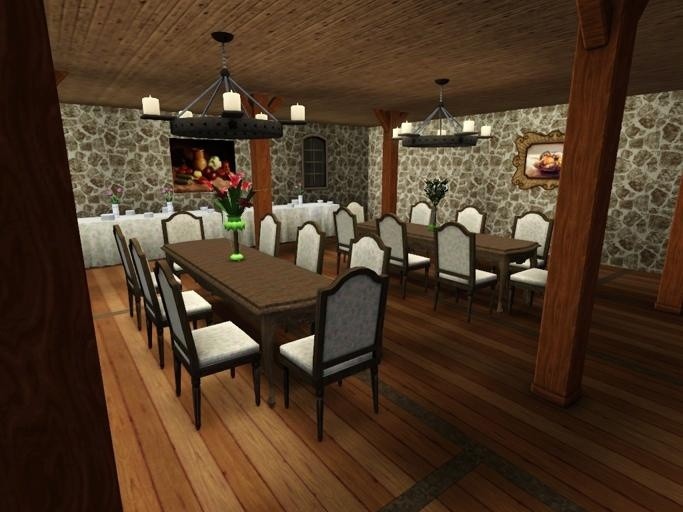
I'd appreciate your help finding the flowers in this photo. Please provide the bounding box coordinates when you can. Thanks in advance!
[161,187,174,202]
[105,187,123,204]
[202,169,256,212]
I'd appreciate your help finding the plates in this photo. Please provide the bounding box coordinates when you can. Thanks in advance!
[327,201,333,204]
[143,212,154,218]
[317,199,323,203]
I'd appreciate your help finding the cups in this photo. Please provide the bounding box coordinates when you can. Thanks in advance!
[125,210,136,216]
[287,203,294,208]
[291,200,299,206]
[162,207,170,219]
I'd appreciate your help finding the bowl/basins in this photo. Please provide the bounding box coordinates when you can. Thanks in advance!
[207,208,215,213]
[200,207,208,212]
[100,213,116,221]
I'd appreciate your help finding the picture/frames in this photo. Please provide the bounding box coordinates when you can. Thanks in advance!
[513,128,566,190]
[169,139,235,193]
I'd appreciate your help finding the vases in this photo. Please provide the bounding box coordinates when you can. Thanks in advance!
[426,179,448,230]
[164,202,175,214]
[106,205,123,218]
[224,215,246,262]
[298,194,303,204]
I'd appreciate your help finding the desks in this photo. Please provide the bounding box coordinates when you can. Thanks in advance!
[77,202,341,269]
[160,238,335,406]
[357,219,539,316]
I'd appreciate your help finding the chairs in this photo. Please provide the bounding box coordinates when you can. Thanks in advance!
[278,267,389,441]
[409,201,434,225]
[341,201,368,223]
[455,205,486,234]
[433,223,491,322]
[155,260,260,429]
[333,207,359,274]
[127,239,211,368]
[347,232,390,277]
[511,212,554,270]
[259,213,281,256]
[113,225,181,333]
[295,221,325,274]
[376,212,431,301]
[508,267,548,315]
[161,211,205,244]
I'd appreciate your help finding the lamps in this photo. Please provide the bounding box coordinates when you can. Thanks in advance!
[391,78,494,147]
[140,32,306,138]
